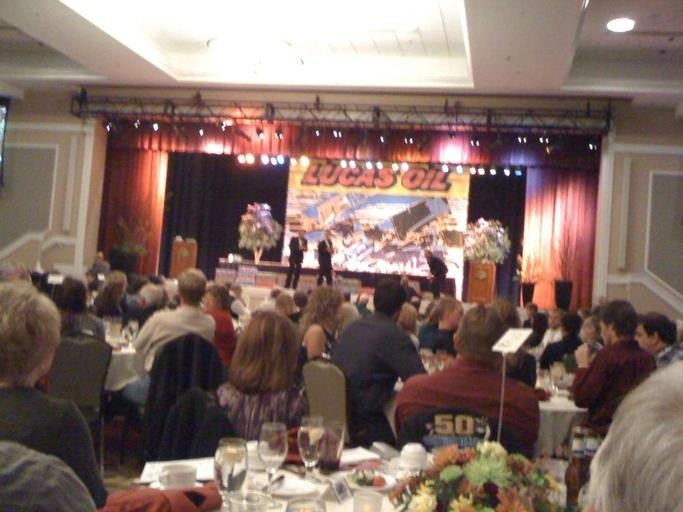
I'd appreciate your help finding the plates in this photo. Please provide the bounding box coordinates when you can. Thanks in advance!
[253,472,319,497]
[327,470,397,491]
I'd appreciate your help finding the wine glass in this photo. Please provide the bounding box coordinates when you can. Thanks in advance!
[105,313,137,350]
[256,421,286,509]
[421,346,447,369]
[214,438,248,506]
[296,414,323,501]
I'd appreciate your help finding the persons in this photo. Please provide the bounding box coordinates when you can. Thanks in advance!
[283,230,310,290]
[0,248,683,512]
[317,230,336,287]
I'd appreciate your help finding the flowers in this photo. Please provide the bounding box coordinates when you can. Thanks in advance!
[107,215,149,258]
[237,203,283,262]
[463,216,512,264]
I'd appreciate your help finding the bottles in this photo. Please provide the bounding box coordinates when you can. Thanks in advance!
[581,429,599,486]
[564,425,584,512]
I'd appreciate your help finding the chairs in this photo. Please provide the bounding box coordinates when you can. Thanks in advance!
[42,333,233,480]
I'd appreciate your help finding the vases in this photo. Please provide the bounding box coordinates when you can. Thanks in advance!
[108,254,141,274]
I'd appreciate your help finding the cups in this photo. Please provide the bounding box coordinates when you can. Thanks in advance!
[399,441,427,471]
[157,466,196,489]
[320,420,344,472]
[285,499,326,512]
[351,489,383,511]
